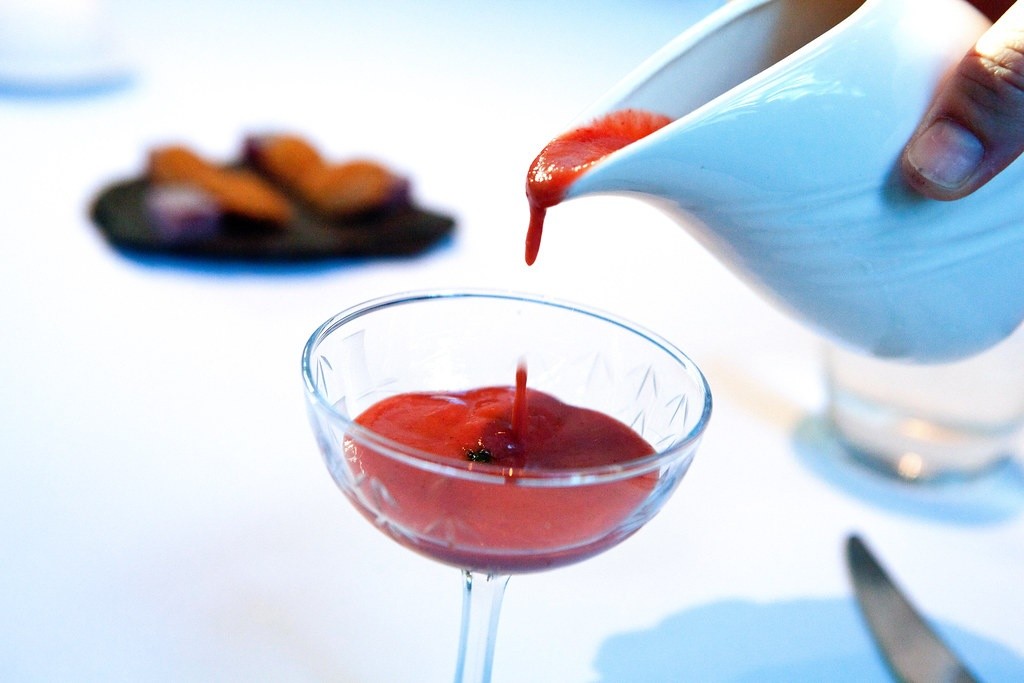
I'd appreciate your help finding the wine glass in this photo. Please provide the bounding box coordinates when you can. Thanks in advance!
[301,290,713,683]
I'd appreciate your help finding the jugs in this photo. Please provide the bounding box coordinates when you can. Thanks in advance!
[526,0,1023,363]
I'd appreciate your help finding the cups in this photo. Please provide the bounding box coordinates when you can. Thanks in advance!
[833,322,1024,479]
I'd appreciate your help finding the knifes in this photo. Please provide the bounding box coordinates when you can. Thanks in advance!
[848,534,974,683]
